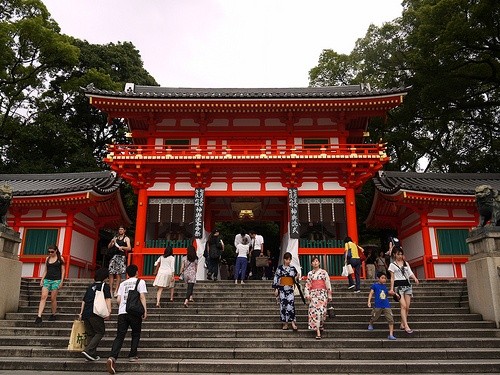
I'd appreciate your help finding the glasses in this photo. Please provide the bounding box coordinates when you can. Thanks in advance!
[48,251,57,253]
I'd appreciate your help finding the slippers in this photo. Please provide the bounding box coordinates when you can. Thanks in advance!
[283,323,324,340]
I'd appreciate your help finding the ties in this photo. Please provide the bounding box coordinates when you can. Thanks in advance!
[253,239,255,251]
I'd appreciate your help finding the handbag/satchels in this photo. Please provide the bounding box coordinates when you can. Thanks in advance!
[67,314,88,352]
[341,265,348,276]
[153,256,160,276]
[126,279,145,316]
[327,301,335,320]
[256,255,269,267]
[179,273,185,281]
[344,242,351,261]
[346,264,354,275]
[216,236,223,250]
[357,245,366,261]
[93,284,109,318]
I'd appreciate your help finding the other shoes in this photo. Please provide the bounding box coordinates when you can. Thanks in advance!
[48,314,57,321]
[368,326,373,330]
[34,317,43,326]
[353,290,360,294]
[405,328,412,333]
[388,335,396,340]
[348,284,355,290]
[85,356,100,361]
[400,326,405,329]
[81,352,95,361]
[129,356,138,361]
[107,358,116,374]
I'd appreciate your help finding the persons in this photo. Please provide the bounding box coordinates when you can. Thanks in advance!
[368,272,400,340]
[78,268,112,360]
[106,264,148,374]
[34,244,66,325]
[343,236,364,294]
[108,226,131,297]
[234,229,275,284]
[153,246,175,308]
[364,235,401,279]
[388,245,419,334]
[272,252,300,331]
[304,256,333,339]
[181,245,199,308]
[204,228,223,280]
